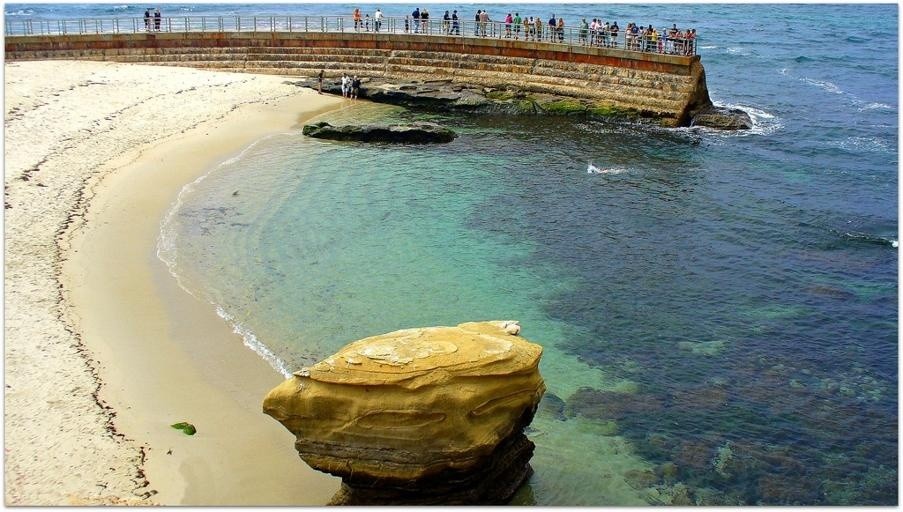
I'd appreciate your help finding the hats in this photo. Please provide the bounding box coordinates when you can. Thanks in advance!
[662,28,666,30]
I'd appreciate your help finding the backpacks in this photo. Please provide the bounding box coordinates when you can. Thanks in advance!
[412,11,417,16]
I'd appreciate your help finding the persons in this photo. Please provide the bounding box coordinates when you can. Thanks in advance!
[144,10,162,32]
[549,14,564,43]
[625,23,696,55]
[505,13,521,40]
[341,73,361,101]
[475,10,491,36]
[444,10,460,35]
[317,70,324,92]
[404,8,429,33]
[580,18,619,48]
[354,9,384,32]
[524,17,541,42]
